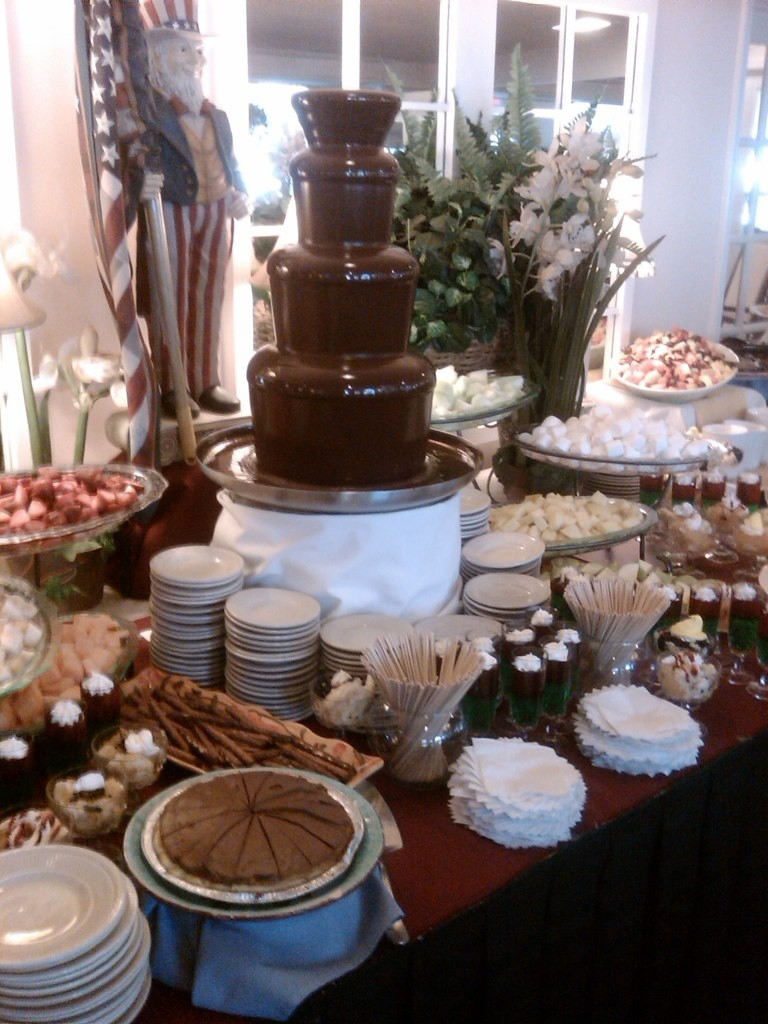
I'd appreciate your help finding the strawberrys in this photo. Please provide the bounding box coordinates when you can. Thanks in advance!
[0,465,145,537]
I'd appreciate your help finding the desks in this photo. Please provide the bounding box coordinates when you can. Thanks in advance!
[0,456,768,1024]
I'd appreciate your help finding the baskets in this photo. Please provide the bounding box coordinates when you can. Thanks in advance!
[425,336,499,376]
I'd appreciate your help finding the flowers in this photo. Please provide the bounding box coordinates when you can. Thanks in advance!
[382,41,666,423]
[1,228,128,469]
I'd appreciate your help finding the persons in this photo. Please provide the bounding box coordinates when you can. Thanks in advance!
[128,0,243,421]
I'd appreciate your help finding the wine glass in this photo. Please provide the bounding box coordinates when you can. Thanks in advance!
[721,584,764,685]
[648,586,683,639]
[502,606,583,750]
[746,604,768,700]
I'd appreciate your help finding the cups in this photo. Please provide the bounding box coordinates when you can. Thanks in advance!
[365,694,471,782]
[663,535,768,582]
[689,583,723,654]
[747,406,768,465]
[702,424,749,472]
[724,419,766,468]
[639,473,762,512]
[577,626,659,699]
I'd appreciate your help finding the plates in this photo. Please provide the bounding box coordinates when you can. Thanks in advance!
[149,489,553,722]
[0,574,60,695]
[512,424,715,476]
[0,845,152,1024]
[613,340,739,403]
[58,611,140,685]
[0,463,169,559]
[429,375,542,431]
[124,767,386,920]
[491,495,659,551]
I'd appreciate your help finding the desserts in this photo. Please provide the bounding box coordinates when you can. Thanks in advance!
[652,614,723,711]
[0,722,166,852]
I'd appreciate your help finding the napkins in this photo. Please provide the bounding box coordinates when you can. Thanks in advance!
[447,684,705,849]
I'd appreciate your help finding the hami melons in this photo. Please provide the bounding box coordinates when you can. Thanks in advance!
[1,612,129,732]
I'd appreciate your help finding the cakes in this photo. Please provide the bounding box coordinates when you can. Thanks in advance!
[151,770,356,892]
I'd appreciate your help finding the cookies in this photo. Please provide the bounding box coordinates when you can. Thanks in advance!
[118,675,355,782]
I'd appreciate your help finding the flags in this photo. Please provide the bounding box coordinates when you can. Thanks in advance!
[70,0,203,475]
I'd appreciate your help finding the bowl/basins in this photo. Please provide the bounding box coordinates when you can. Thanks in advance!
[46,764,128,838]
[91,724,168,791]
[657,651,722,709]
